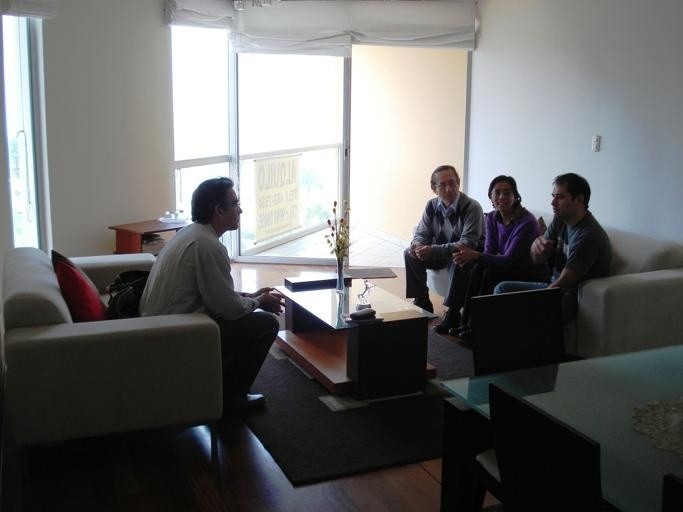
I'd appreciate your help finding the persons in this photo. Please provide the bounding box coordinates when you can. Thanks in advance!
[139,177,289,416]
[402,166,486,333]
[448,175,552,343]
[494,172,614,363]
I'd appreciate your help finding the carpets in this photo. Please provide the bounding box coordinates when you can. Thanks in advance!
[243,329,505,488]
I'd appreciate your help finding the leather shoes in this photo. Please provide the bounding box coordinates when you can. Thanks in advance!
[447,325,466,338]
[458,329,473,339]
[225,394,265,416]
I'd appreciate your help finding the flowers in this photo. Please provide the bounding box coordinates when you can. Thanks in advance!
[325,200,353,259]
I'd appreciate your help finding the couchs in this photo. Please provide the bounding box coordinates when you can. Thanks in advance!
[429,212,682,358]
[1,248,223,480]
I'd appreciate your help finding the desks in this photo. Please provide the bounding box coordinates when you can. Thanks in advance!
[440,345,683,511]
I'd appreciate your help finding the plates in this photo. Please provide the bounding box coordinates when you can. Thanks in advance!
[158,218,186,223]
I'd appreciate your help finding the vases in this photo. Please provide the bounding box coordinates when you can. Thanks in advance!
[334,258,347,325]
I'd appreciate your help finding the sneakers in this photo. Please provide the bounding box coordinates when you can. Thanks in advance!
[412,299,434,315]
[432,311,459,332]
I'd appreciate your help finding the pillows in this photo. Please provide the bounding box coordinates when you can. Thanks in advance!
[51,250,108,322]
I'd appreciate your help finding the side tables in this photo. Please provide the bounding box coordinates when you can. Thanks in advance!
[108,220,186,254]
